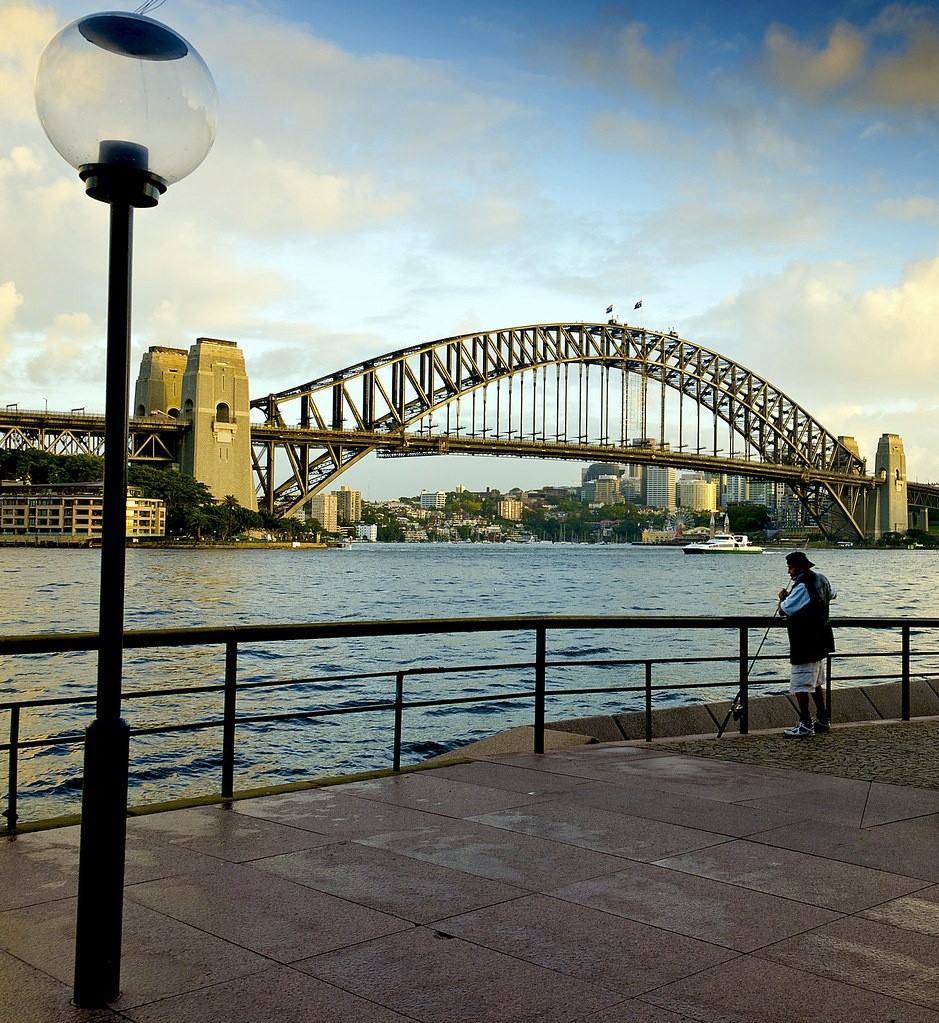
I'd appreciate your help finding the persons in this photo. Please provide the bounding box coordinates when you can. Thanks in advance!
[777,551,837,736]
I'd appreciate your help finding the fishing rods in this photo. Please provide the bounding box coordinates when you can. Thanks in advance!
[715,539,810,741]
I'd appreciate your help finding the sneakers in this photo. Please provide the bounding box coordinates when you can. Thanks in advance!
[783,722,816,737]
[812,717,831,734]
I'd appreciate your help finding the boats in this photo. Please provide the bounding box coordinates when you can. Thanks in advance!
[682,532,764,555]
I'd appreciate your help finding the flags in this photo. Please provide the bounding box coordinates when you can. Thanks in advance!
[606,305,612,313]
[633,300,641,309]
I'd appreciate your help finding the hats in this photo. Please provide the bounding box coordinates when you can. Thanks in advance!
[785,551,815,568]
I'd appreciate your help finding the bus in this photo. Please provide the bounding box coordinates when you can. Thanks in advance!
[836,541,853,547]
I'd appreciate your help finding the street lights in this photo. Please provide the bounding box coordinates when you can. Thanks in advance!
[37,9,219,1008]
